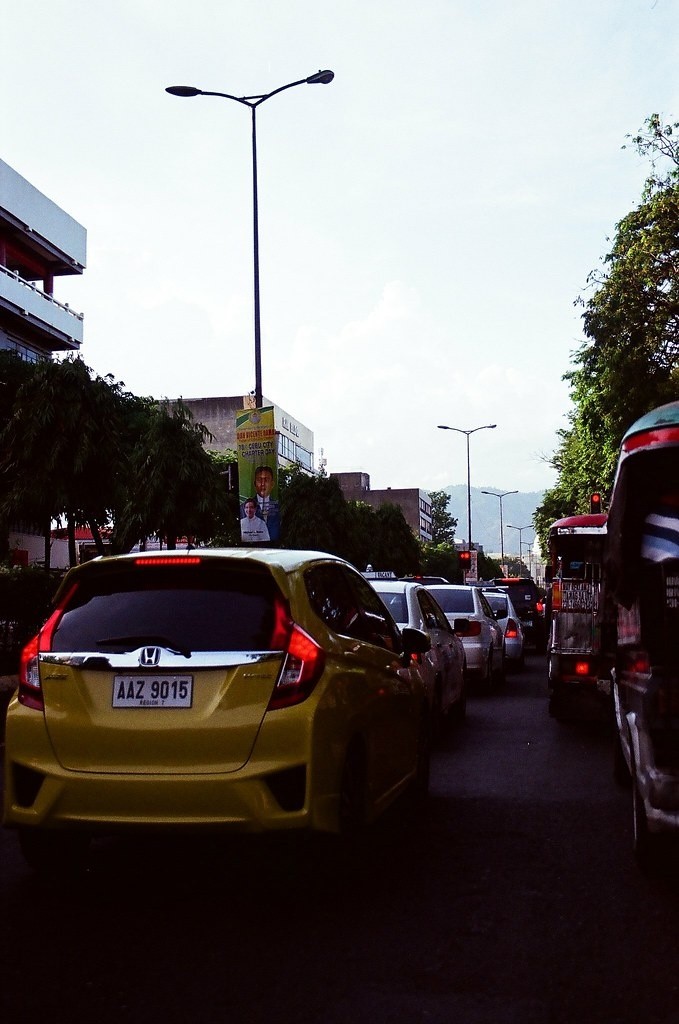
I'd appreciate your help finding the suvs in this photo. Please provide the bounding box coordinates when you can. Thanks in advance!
[609,402,679,854]
[489,579,548,653]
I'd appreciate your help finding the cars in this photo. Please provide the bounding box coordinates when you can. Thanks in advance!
[422,584,506,694]
[482,592,525,676]
[2,546,431,882]
[360,565,467,738]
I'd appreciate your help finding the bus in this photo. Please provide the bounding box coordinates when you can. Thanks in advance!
[547,514,610,718]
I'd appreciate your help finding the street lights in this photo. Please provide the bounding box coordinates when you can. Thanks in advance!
[164,70,336,409]
[482,491,519,577]
[507,526,534,577]
[526,542,534,578]
[439,425,496,550]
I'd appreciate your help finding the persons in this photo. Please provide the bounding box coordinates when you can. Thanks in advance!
[240,466,280,542]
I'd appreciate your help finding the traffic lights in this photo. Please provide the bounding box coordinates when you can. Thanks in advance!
[464,552,471,569]
[458,551,463,567]
[591,493,601,514]
